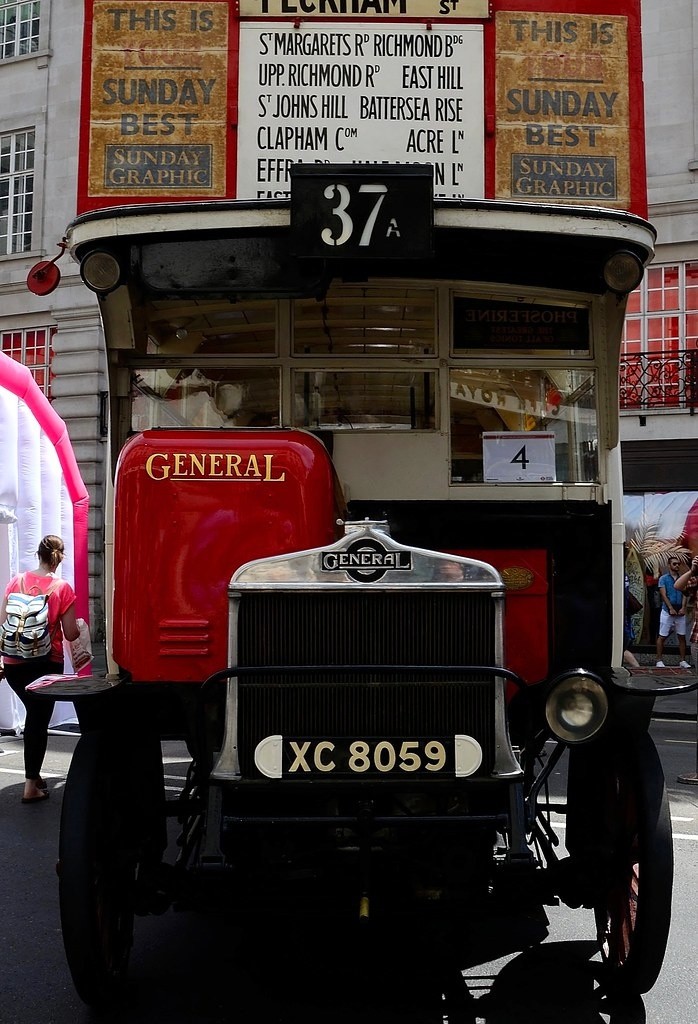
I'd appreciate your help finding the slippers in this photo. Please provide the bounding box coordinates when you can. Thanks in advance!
[21,776,48,805]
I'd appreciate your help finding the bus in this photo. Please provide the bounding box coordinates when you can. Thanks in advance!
[33,156,691,1024]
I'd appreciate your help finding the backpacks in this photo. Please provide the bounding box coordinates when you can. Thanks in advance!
[3,594,51,660]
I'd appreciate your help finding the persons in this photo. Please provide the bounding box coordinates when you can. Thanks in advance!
[623,574,641,669]
[0,535,80,805]
[656,557,691,669]
[674,556,698,674]
